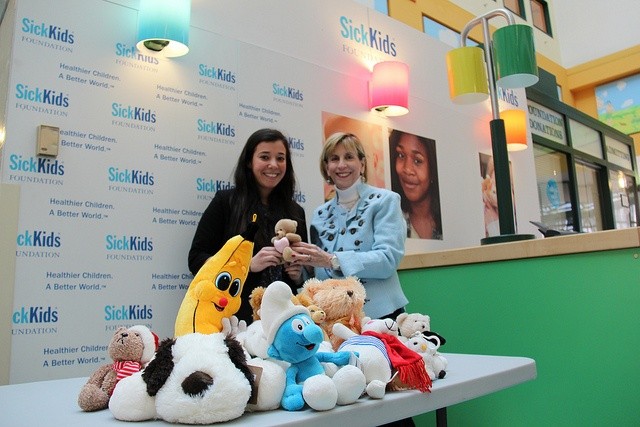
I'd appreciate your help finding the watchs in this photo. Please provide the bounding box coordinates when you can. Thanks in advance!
[331,252,340,271]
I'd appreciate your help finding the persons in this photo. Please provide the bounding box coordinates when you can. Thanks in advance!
[187,127,309,327]
[479,152,518,238]
[388,128,444,240]
[291,130,409,321]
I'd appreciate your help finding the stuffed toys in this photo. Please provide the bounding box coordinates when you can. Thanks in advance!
[174,221,260,338]
[291,293,330,342]
[270,218,301,263]
[331,317,399,400]
[396,312,448,379]
[77,325,159,413]
[303,276,367,353]
[109,333,287,425]
[260,280,367,412]
[397,330,447,381]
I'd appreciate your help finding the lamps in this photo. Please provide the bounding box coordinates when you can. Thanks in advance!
[136,0,191,58]
[448,8,541,246]
[500,109,527,151]
[370,60,409,117]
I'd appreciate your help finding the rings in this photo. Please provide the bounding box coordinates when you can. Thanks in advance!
[308,255,311,261]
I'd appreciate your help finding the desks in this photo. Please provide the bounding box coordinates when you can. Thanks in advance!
[0,353,537,427]
